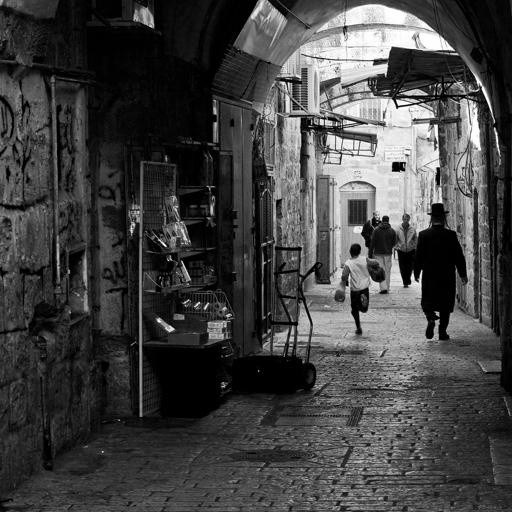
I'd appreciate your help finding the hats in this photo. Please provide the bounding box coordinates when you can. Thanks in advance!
[426,203,449,214]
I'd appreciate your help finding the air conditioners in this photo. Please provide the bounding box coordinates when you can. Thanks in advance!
[289,65,324,118]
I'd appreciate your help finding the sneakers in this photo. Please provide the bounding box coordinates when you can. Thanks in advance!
[361,294,368,307]
[378,276,419,295]
[439,334,449,340]
[426,320,436,339]
[355,329,362,335]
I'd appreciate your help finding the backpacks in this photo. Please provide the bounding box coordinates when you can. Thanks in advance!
[366,258,386,283]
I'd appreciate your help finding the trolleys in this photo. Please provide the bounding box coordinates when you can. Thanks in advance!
[232,246,324,393]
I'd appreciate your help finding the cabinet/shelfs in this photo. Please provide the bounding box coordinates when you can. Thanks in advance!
[123,136,238,420]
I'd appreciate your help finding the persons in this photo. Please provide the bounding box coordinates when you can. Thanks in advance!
[361,211,381,249]
[340,243,378,336]
[367,215,396,295]
[414,203,470,341]
[394,214,417,288]
